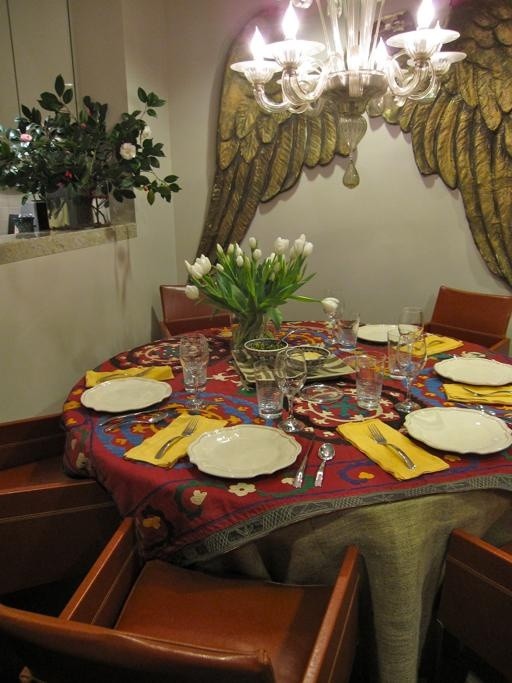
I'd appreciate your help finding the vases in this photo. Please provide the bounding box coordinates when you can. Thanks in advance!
[64,194,94,227]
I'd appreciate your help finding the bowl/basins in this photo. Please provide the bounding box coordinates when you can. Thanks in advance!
[244,338,289,366]
[285,345,330,374]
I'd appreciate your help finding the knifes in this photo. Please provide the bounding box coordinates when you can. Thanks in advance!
[97,405,177,427]
[292,429,318,488]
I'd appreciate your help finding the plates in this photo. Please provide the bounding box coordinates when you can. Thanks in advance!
[186,424,300,482]
[402,406,512,456]
[231,342,355,385]
[78,375,173,415]
[429,353,512,387]
[354,323,417,345]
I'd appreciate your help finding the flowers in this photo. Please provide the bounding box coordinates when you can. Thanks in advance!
[182,233,340,335]
[0,74,183,218]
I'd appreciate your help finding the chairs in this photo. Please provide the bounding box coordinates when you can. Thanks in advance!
[1,515,362,682]
[156,284,235,337]
[423,285,511,354]
[1,411,118,597]
[434,531,511,682]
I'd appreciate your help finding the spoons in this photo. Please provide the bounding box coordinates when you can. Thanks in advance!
[104,413,169,432]
[313,441,336,488]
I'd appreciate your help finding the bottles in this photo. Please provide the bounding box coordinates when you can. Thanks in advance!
[323,308,338,344]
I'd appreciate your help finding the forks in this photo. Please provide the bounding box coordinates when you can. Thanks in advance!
[94,365,155,383]
[366,420,416,470]
[152,419,201,461]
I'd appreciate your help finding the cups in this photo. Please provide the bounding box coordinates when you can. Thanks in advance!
[252,358,285,420]
[398,305,426,343]
[353,349,383,412]
[386,329,411,381]
[182,337,209,392]
[337,310,360,350]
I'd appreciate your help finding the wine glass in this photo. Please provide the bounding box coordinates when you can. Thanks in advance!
[179,332,210,410]
[273,347,310,433]
[398,333,427,415]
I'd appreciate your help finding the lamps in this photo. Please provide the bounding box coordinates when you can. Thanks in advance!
[230,1,469,114]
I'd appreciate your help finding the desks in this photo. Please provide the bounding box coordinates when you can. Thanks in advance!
[63,321,512,521]
